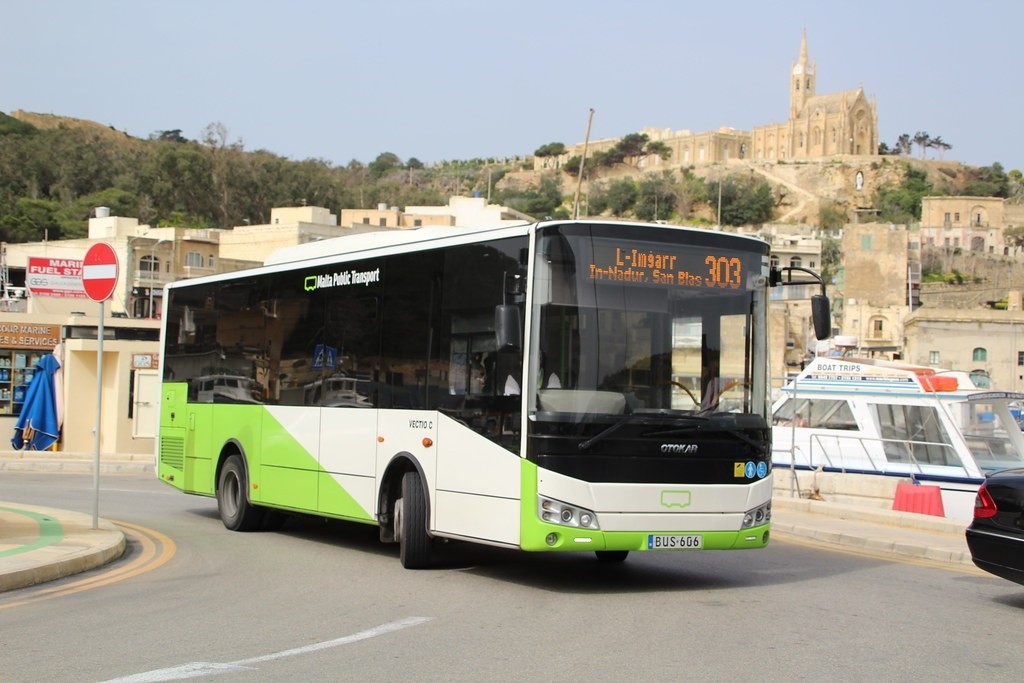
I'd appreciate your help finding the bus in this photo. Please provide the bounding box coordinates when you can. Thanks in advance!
[153,219,830,570]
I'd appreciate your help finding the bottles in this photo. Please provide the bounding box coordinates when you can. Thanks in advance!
[0,359,11,399]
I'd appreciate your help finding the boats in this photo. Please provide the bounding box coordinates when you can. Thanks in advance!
[196,375,266,406]
[302,378,374,409]
[774,354,1024,522]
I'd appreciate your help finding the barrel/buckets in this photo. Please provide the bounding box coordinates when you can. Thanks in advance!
[15,354,25,367]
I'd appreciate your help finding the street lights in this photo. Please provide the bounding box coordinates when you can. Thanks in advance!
[301,198,307,207]
[149,238,167,318]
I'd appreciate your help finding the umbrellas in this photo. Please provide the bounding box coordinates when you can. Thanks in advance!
[11,354,61,451]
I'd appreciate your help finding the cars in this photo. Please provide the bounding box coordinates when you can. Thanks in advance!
[964,468,1024,586]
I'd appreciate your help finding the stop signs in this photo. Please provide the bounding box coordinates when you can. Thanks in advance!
[81,242,120,303]
